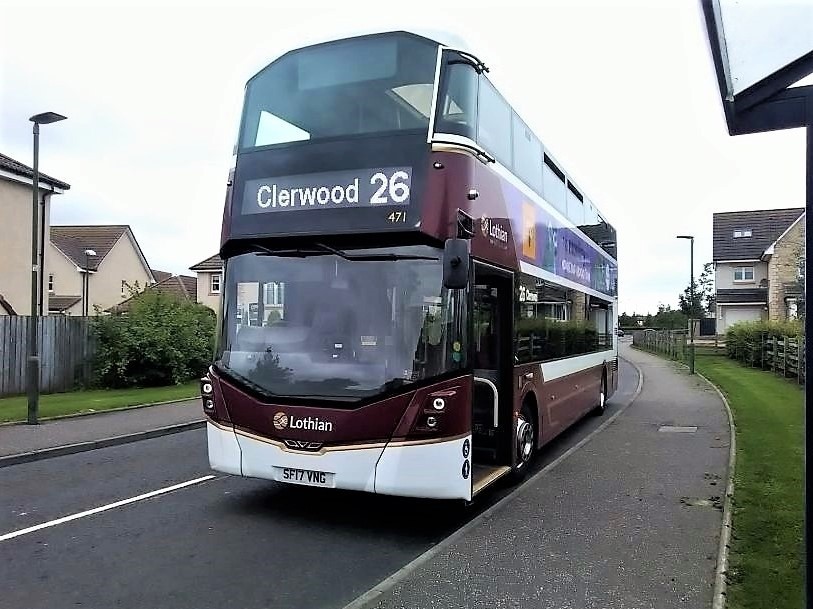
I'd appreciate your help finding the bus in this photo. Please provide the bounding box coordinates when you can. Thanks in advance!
[197,28,619,509]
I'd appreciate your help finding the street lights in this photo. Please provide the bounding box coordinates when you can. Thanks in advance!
[84,249,96,317]
[26,111,69,426]
[676,235,695,374]
